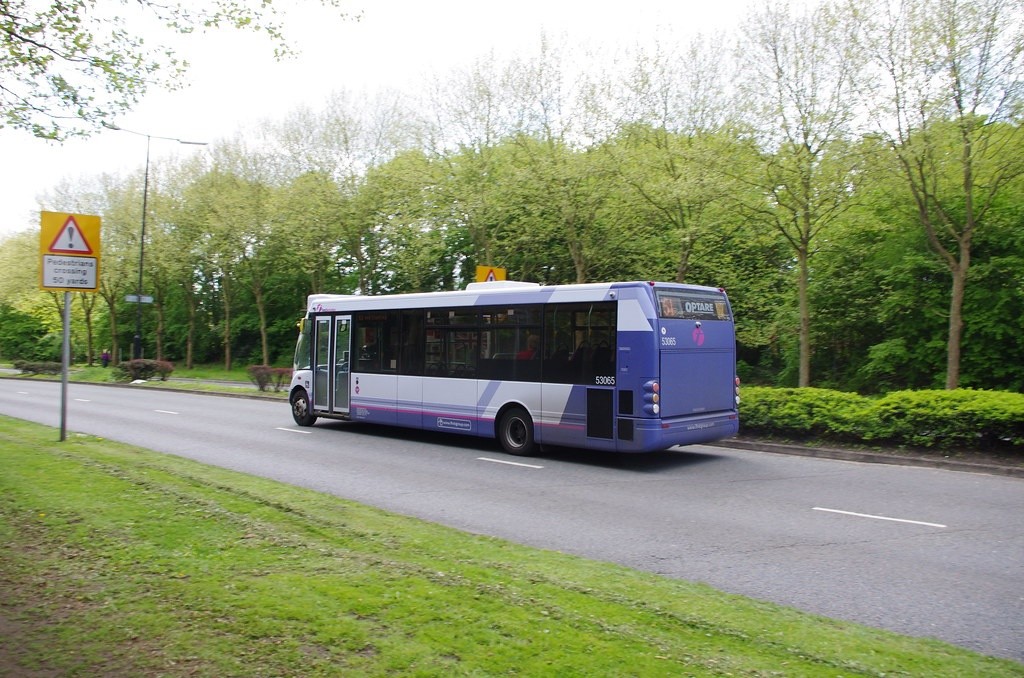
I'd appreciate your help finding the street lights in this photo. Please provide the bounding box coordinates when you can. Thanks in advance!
[102,121,209,363]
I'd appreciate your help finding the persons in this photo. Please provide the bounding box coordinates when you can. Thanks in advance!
[517,335,541,360]
[362,317,392,369]
[102,350,111,368]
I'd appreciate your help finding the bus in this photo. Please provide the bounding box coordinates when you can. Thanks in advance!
[289,281,741,457]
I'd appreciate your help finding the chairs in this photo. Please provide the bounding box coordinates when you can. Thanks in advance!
[425,340,611,381]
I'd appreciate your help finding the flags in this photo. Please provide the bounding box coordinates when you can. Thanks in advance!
[455,331,487,351]
